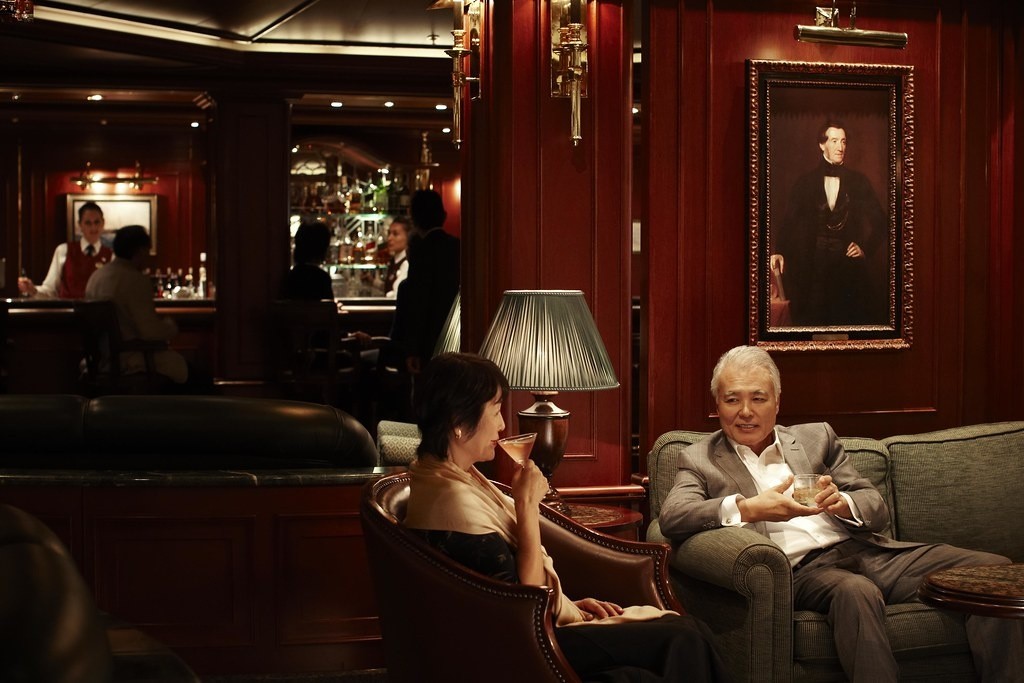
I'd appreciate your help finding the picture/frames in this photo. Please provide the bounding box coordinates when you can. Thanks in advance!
[744,58,915,357]
[66,192,158,256]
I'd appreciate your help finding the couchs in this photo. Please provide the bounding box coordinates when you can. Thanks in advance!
[0,391,378,478]
[648,420,1024,683]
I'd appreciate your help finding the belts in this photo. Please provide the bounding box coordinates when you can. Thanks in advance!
[792,537,853,573]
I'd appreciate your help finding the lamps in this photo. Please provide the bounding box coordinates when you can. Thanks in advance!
[793,0,909,49]
[477,288,620,518]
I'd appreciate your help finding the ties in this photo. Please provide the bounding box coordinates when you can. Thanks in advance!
[86,245,95,256]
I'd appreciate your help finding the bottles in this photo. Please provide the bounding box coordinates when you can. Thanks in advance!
[291,172,412,297]
[19,268,30,298]
[145,252,215,298]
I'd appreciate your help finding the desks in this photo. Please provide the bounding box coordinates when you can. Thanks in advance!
[566,503,643,542]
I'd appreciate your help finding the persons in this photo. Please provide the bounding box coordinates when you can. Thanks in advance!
[284,222,373,371]
[376,189,460,384]
[770,121,882,295]
[17,202,117,299]
[83,225,179,340]
[401,352,739,683]
[658,344,1023,682]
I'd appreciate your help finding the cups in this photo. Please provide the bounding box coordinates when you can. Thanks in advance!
[794,474,822,506]
[497,432,537,469]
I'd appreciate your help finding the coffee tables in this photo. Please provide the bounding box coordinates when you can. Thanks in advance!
[915,564,1024,618]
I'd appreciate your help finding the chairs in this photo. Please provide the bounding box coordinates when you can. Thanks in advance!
[272,299,392,446]
[0,297,172,395]
[360,472,687,683]
[1,502,201,682]
